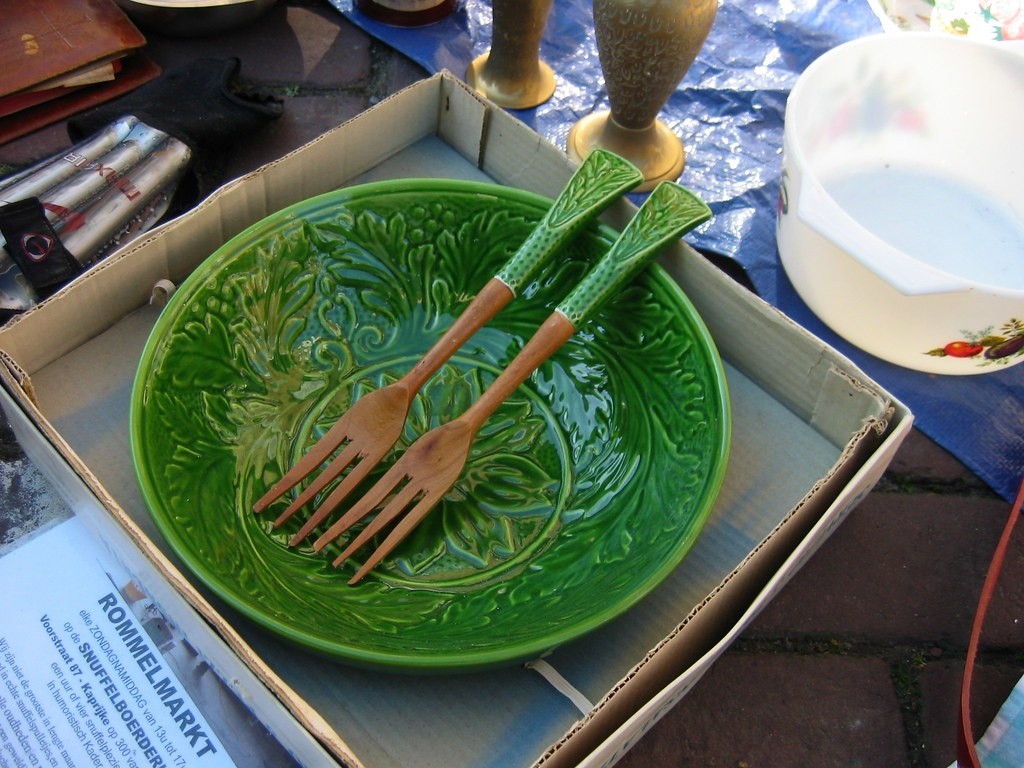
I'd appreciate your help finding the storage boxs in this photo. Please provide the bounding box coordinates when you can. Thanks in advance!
[0,69,916,768]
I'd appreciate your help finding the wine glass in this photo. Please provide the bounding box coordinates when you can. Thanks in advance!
[569,2,723,191]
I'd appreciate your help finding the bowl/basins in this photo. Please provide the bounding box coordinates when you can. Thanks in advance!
[771,31,1024,375]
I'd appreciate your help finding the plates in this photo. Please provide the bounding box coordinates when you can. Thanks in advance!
[129,179,733,672]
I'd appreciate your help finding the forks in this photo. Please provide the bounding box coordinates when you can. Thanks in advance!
[251,145,713,589]
